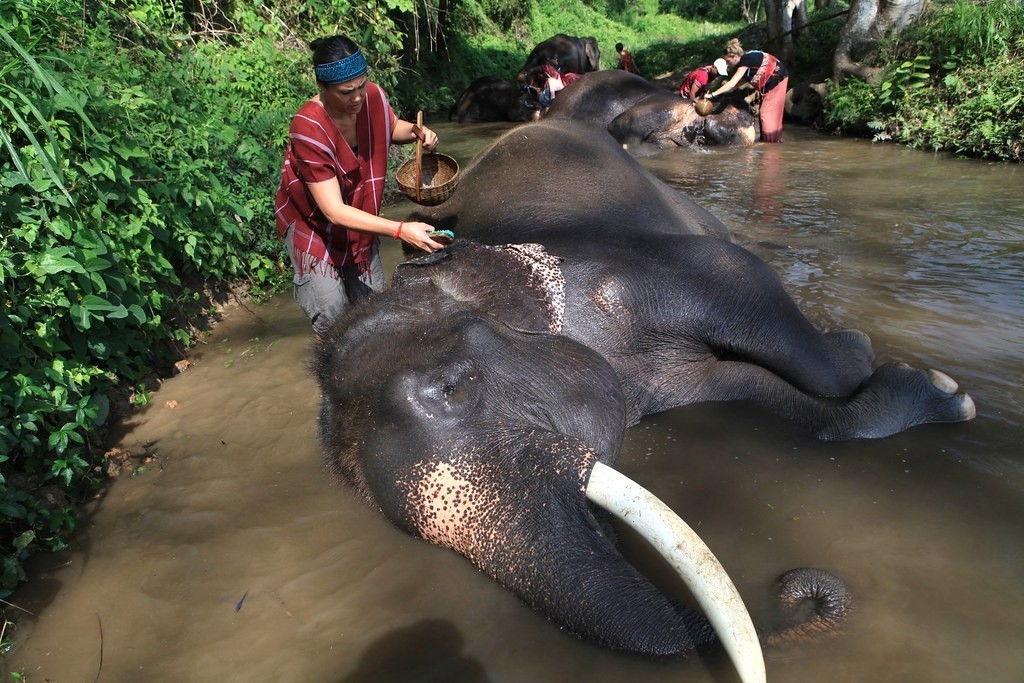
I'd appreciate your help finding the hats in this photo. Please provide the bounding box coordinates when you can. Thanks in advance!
[714,58,728,76]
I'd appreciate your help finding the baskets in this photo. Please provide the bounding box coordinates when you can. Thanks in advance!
[395,111,459,207]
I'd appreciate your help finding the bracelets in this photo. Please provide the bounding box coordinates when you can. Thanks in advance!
[393,221,403,240]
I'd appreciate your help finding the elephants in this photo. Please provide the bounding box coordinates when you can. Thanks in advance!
[302,117,981,683]
[449,33,762,154]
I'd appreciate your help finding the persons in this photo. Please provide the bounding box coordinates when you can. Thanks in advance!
[680,58,729,100]
[518,64,583,114]
[616,43,634,73]
[704,38,789,143]
[276,36,448,338]
